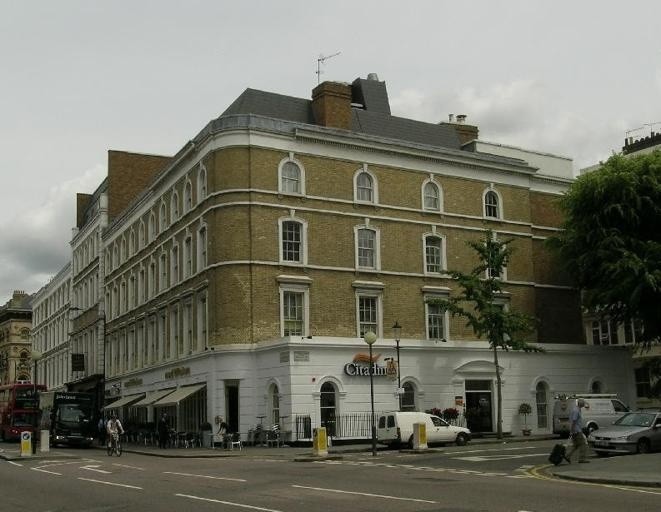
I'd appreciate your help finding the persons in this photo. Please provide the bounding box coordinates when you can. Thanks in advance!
[217,416,233,449]
[158,413,172,449]
[107,414,125,454]
[96,418,108,446]
[565,398,592,463]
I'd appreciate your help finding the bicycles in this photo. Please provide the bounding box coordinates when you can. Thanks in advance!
[105,431,124,457]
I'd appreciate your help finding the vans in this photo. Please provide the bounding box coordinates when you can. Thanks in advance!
[552,393,635,436]
[376,411,473,447]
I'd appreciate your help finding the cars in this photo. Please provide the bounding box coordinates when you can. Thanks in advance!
[587,410,661,455]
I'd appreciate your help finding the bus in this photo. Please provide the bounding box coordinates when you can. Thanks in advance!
[1,384,47,440]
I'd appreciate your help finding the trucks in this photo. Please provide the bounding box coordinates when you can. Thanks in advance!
[37,391,96,447]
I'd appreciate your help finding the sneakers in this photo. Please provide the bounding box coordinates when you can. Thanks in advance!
[579,458,591,463]
[564,452,572,463]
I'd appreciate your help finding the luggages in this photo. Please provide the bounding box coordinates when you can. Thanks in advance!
[550,434,573,465]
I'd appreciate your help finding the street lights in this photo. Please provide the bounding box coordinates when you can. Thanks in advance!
[390,319,404,409]
[68,305,107,447]
[31,349,43,456]
[363,330,380,455]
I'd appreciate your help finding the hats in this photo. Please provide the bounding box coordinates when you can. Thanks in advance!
[578,398,586,403]
